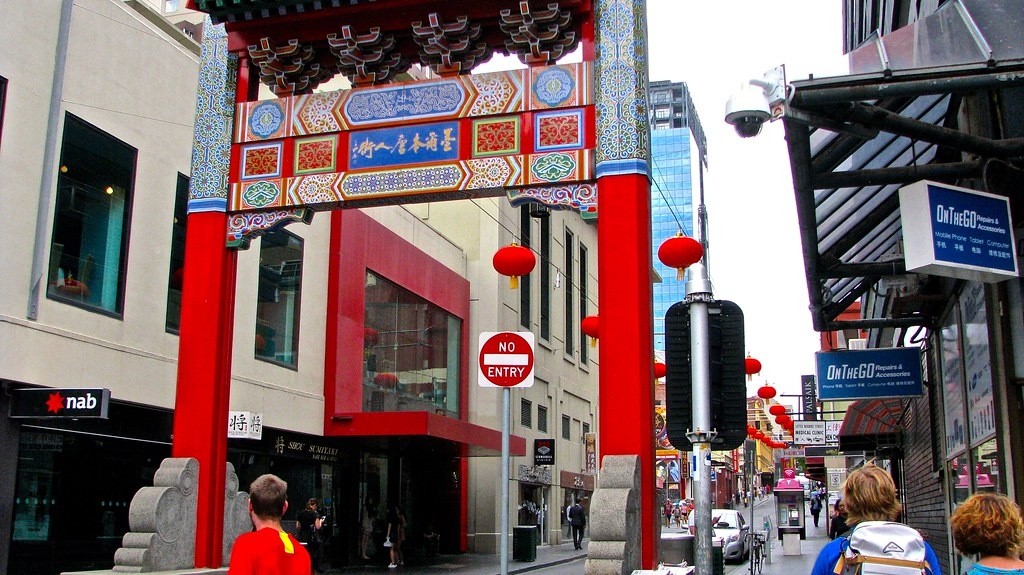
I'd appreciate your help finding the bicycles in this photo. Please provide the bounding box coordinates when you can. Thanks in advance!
[744,532,767,575]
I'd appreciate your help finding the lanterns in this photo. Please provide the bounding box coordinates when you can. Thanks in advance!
[658,233,703,281]
[745,356,793,449]
[375,374,398,390]
[256,335,265,350]
[493,243,536,289]
[654,360,666,385]
[363,327,379,351]
[582,314,600,347]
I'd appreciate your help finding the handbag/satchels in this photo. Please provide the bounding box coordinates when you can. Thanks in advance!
[811,510,814,515]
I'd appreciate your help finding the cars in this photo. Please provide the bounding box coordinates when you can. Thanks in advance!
[682,509,751,564]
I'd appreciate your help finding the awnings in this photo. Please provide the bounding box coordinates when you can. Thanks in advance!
[836,398,905,452]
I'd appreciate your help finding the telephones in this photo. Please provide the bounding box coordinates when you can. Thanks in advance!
[789,507,798,520]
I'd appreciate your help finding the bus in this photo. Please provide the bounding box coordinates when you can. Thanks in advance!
[761,472,774,489]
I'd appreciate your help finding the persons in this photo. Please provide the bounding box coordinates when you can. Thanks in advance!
[57,268,82,295]
[664,498,695,528]
[360,495,375,559]
[569,498,586,550]
[227,474,311,575]
[951,493,1024,575]
[535,502,545,541]
[828,499,851,542]
[296,498,333,575]
[811,495,822,527]
[811,465,942,575]
[566,500,573,537]
[736,483,770,503]
[812,481,826,500]
[386,505,406,568]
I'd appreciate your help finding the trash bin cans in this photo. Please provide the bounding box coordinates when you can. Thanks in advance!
[513,526,537,561]
[712,537,726,575]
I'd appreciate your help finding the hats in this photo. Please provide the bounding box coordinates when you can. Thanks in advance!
[691,498,694,501]
[682,500,686,504]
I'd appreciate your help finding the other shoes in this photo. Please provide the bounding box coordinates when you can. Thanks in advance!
[577,545,583,549]
[575,547,578,550]
[815,524,818,527]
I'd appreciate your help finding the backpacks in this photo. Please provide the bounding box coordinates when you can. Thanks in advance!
[833,521,933,575]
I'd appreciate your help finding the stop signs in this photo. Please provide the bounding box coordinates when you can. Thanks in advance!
[477,331,535,388]
